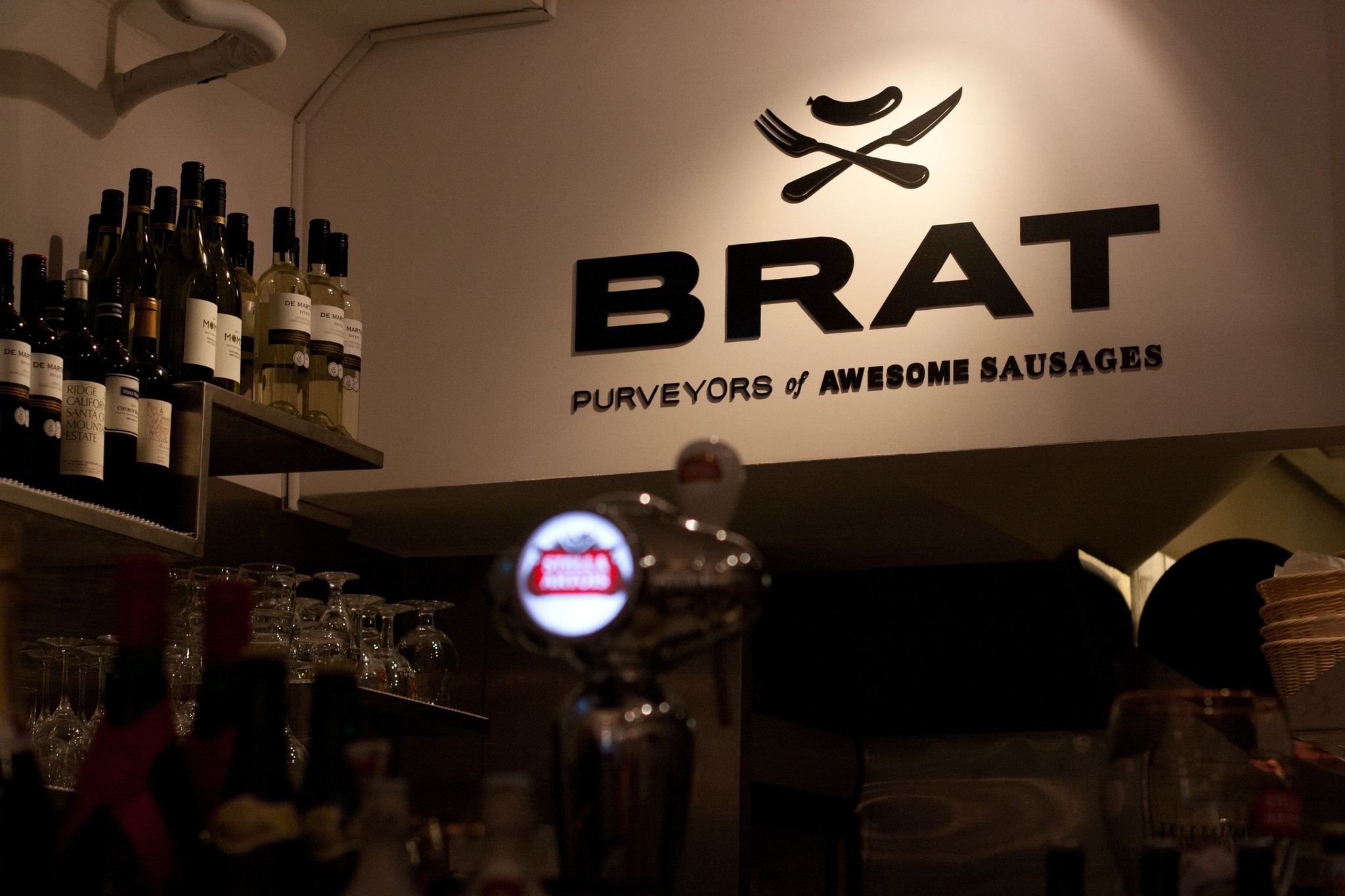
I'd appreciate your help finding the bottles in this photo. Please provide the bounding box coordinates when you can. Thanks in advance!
[1,153,370,547]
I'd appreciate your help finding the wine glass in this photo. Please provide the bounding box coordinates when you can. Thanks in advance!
[0,554,473,834]
